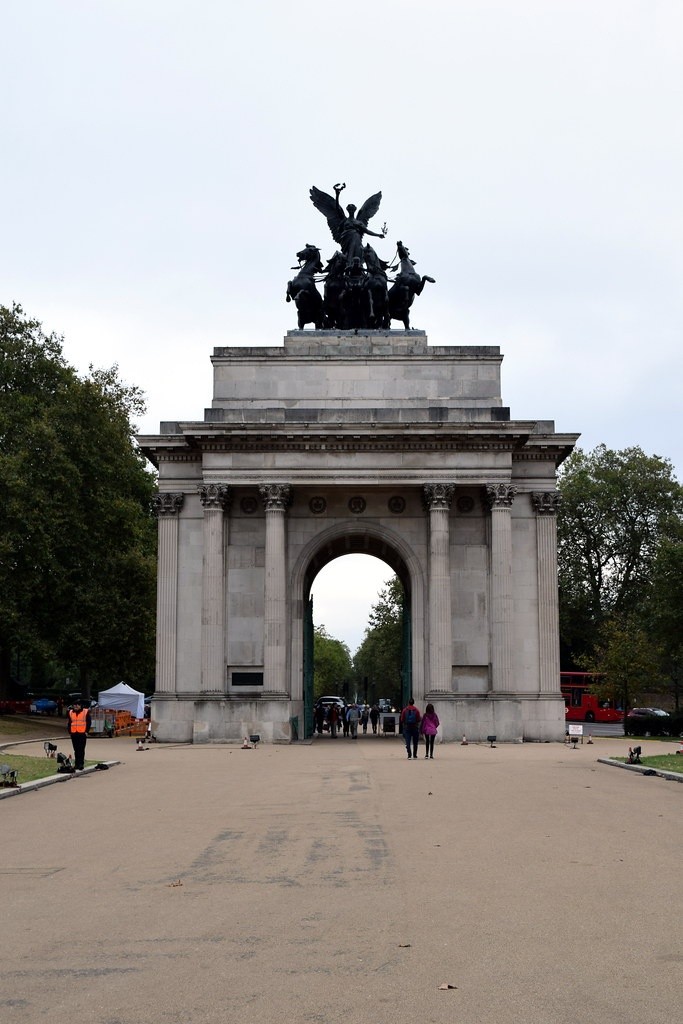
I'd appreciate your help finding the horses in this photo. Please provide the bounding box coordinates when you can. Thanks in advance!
[286,241,436,332]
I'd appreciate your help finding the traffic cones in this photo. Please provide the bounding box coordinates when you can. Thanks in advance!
[241,737,251,749]
[461,734,468,745]
[136,740,144,751]
[587,734,593,744]
[625,747,633,763]
[680,741,683,755]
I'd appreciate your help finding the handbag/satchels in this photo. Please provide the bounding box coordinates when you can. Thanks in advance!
[359,719,362,725]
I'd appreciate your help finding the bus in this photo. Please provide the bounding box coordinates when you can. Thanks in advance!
[560,672,625,722]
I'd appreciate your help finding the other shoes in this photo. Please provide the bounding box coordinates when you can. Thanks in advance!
[425,756,428,759]
[407,757,411,760]
[343,733,345,737]
[374,731,376,734]
[413,755,417,758]
[78,766,84,770]
[373,731,374,734]
[347,734,349,737]
[73,766,79,769]
[430,757,433,759]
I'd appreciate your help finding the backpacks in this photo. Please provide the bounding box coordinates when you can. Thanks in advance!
[404,707,416,724]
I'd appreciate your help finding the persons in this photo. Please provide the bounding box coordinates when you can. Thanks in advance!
[401,699,421,760]
[67,699,91,770]
[335,191,386,301]
[313,702,397,739]
[421,704,440,759]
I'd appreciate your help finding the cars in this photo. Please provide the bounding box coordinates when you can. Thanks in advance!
[623,707,671,737]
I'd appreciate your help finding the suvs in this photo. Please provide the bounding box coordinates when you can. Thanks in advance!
[378,699,392,713]
[314,696,348,710]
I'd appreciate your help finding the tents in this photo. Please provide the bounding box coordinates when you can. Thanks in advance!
[98,681,144,718]
